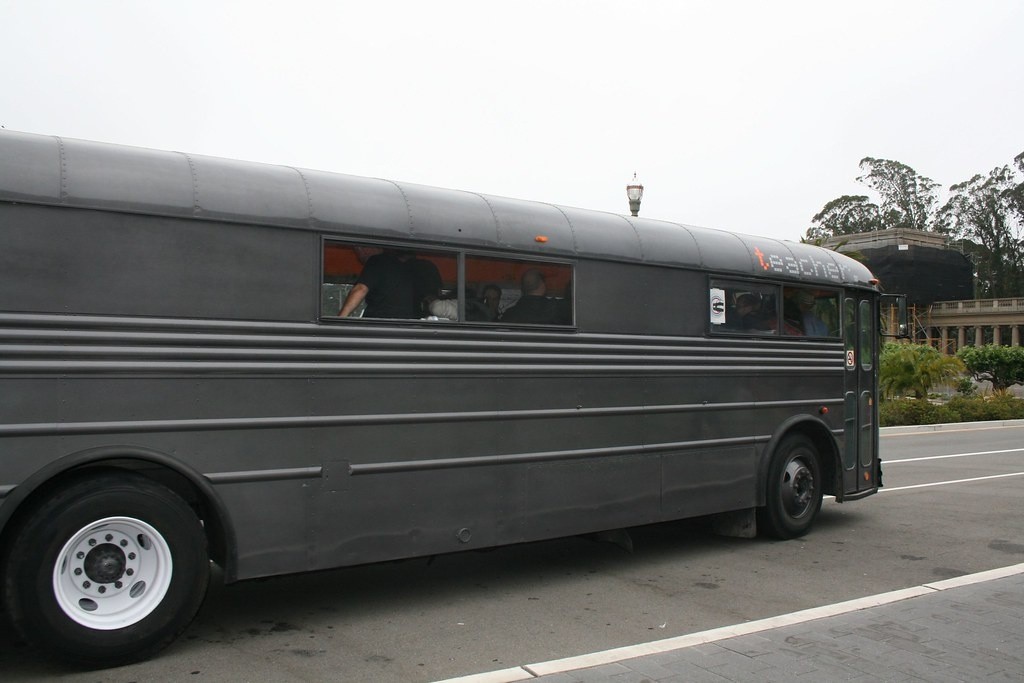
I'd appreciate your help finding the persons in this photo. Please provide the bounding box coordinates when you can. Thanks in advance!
[712,278,839,336]
[340,246,573,325]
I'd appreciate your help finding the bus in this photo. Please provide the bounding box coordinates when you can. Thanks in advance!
[0,125,911,675]
